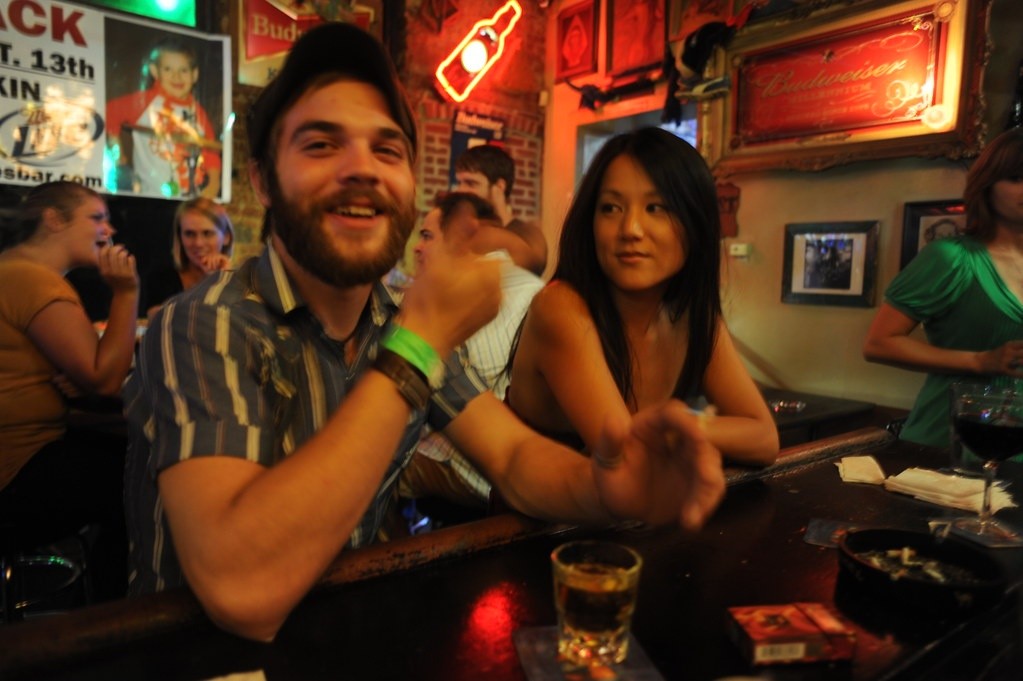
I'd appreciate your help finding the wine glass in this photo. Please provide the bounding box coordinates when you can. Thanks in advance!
[950,378,1023,542]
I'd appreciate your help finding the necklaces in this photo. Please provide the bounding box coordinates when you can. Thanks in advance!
[344,319,361,345]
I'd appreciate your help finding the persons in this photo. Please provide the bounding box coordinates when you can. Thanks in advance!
[108,44,223,199]
[120,21,724,642]
[862,125,1023,463]
[0,144,548,602]
[488,125,782,515]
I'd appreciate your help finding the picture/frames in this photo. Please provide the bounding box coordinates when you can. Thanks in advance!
[780,220,881,308]
[900,196,969,273]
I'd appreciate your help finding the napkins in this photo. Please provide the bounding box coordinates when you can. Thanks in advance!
[832,454,1018,516]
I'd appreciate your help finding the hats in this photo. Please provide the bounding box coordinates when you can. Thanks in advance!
[245,18,418,159]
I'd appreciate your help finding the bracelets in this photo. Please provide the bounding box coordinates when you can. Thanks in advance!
[371,351,432,413]
[377,321,446,390]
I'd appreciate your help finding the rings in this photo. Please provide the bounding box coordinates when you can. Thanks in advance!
[593,450,623,468]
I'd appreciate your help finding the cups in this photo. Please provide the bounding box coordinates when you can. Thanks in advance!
[550,540,644,670]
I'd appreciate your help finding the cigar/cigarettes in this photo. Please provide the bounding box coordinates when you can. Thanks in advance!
[665,404,717,449]
[106,237,113,253]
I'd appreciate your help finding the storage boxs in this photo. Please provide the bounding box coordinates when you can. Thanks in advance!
[722,599,859,667]
